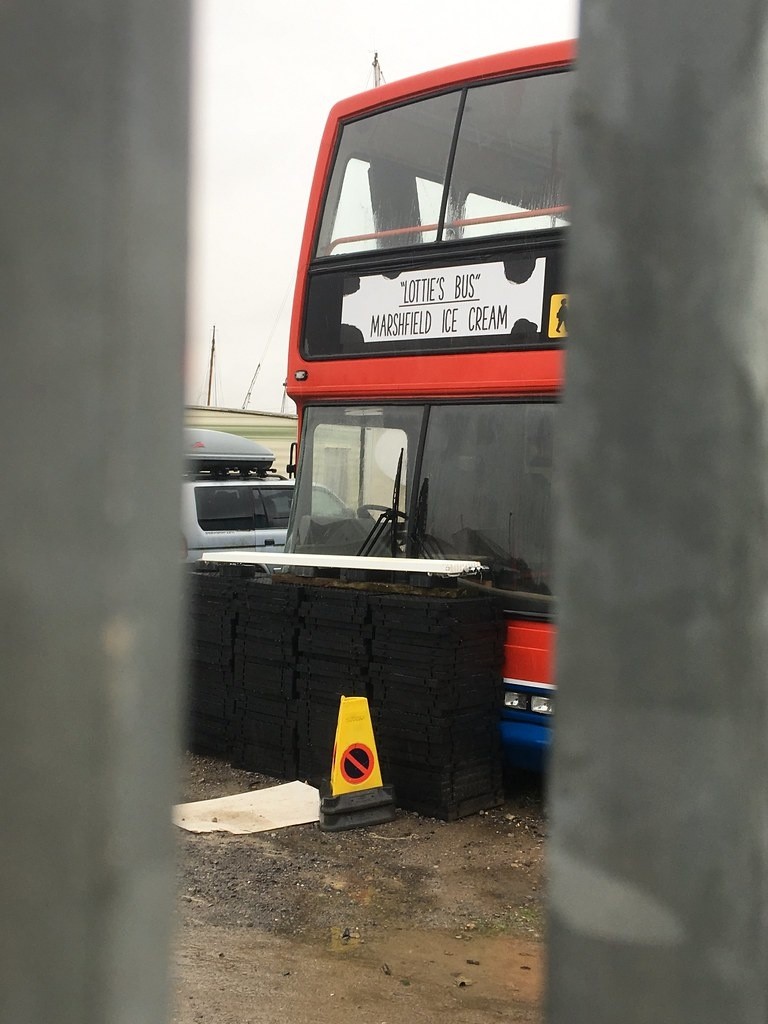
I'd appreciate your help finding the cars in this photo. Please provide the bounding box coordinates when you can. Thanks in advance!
[179,428,401,589]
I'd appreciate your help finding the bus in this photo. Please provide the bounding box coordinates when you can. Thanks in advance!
[276,36,583,775]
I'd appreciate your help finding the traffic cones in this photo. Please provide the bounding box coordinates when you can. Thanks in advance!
[316,696,396,831]
